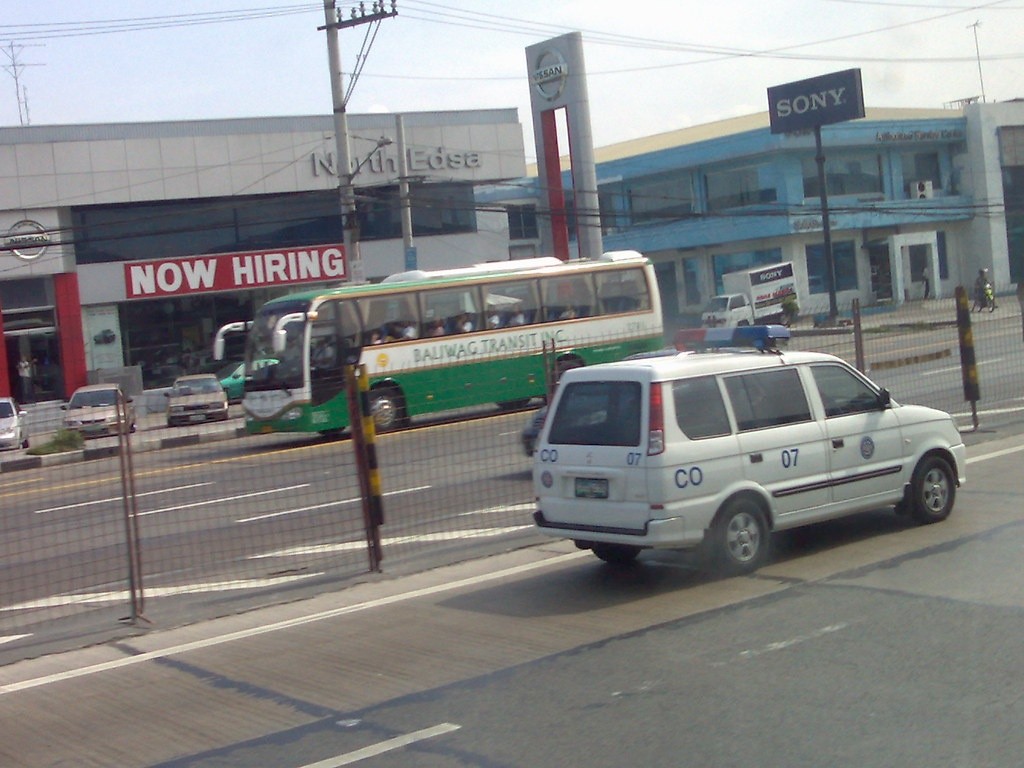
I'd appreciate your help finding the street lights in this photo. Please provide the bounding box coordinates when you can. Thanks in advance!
[337,135,393,283]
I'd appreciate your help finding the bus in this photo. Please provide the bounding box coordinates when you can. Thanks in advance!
[213,248,664,437]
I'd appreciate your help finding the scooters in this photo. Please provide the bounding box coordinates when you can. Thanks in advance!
[975,282,995,314]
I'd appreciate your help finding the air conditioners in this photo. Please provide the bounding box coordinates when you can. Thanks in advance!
[910,181,933,200]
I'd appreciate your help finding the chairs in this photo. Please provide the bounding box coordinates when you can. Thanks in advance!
[178,387,191,396]
[684,383,739,431]
[202,384,216,394]
[371,294,582,343]
[773,376,828,417]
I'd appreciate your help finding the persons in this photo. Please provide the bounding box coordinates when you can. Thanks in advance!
[311,303,615,370]
[390,317,418,341]
[923,266,930,298]
[16,355,38,403]
[453,314,473,333]
[971,267,999,312]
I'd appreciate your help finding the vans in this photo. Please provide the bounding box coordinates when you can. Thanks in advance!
[529,324,970,578]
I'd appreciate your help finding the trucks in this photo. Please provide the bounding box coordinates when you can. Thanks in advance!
[699,258,800,325]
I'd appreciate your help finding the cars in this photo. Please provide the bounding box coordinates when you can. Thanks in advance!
[0,396,31,452]
[519,346,682,459]
[204,358,282,405]
[163,373,230,427]
[60,382,137,440]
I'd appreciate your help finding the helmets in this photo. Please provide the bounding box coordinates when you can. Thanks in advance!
[978,267,988,277]
